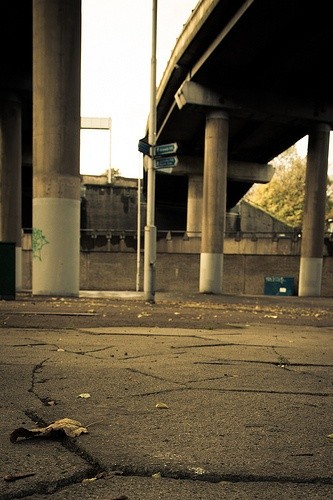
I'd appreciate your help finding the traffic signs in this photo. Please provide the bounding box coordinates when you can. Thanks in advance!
[154,143,178,156]
[152,157,179,169]
[138,140,152,158]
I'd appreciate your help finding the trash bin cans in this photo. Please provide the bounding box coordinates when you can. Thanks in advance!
[263,275,295,296]
